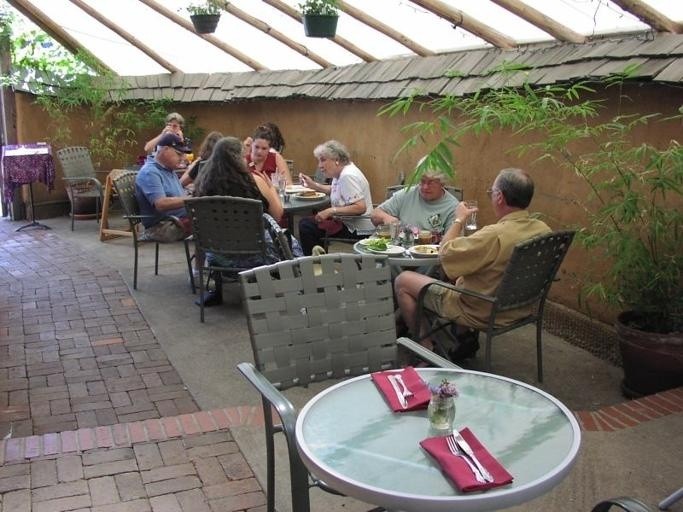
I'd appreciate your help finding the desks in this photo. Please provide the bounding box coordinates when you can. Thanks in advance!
[1,140,54,234]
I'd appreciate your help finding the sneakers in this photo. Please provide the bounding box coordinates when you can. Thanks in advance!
[188,267,218,287]
[195,290,223,307]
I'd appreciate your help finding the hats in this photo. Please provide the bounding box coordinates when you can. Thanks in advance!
[157,132,192,154]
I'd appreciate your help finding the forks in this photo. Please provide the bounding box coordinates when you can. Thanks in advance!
[394,374,413,397]
[444,434,487,484]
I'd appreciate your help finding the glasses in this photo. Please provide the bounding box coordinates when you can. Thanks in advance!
[486,188,499,199]
[167,123,182,129]
[419,178,441,185]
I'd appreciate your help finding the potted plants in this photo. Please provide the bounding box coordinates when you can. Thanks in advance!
[176,1,229,36]
[375,55,681,401]
[293,0,340,39]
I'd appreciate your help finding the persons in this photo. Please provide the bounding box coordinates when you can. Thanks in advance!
[298,140,377,255]
[134,112,305,282]
[393,168,554,367]
[371,170,465,276]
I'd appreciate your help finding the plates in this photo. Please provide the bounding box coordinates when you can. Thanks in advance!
[285,185,326,200]
[359,238,380,245]
[366,246,405,256]
[408,245,440,257]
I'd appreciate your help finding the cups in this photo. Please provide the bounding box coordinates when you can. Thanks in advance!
[377,221,438,248]
[463,201,478,231]
[271,173,279,186]
[278,181,285,192]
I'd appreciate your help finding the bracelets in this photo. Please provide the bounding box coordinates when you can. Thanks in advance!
[333,207,336,216]
[452,218,463,230]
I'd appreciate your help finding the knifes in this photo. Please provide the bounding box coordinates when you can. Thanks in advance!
[387,374,408,409]
[452,428,494,483]
